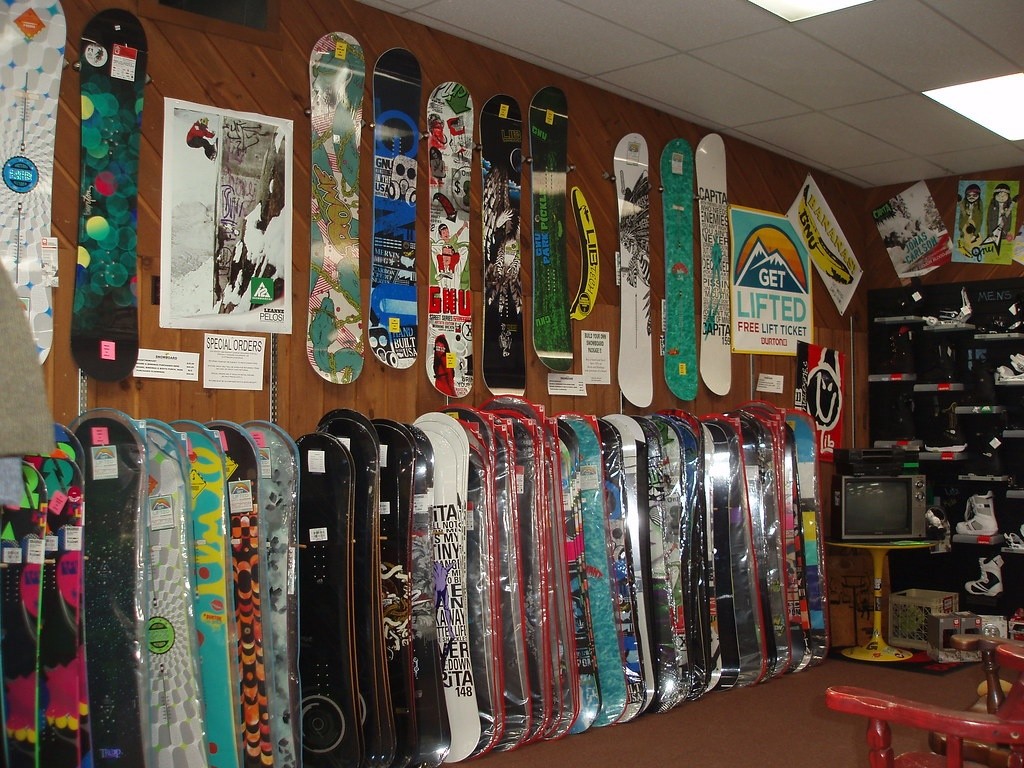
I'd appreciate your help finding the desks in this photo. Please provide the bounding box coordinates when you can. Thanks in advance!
[825,541,939,661]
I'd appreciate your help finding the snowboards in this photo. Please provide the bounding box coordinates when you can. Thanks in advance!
[477,92,527,397]
[72,9,149,382]
[613,132,655,407]
[0,0,66,365]
[530,86,575,371]
[426,81,475,400]
[657,137,702,401]
[696,132,733,396]
[305,32,367,385]
[369,46,422,371]
[0,398,831,768]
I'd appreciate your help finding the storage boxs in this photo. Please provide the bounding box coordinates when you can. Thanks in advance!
[888,589,959,651]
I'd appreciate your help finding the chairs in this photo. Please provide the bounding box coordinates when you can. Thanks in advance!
[824,649,1024,768]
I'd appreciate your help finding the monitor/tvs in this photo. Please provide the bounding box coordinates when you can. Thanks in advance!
[838,474,926,543]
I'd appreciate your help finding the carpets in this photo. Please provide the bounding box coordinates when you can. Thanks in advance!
[828,645,979,676]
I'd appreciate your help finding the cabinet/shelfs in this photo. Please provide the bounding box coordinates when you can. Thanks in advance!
[867,315,1024,607]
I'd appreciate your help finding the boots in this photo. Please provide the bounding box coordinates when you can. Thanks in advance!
[956,491,998,535]
[970,424,1004,475]
[925,399,968,452]
[876,399,919,440]
[965,554,1004,598]
[872,330,915,374]
